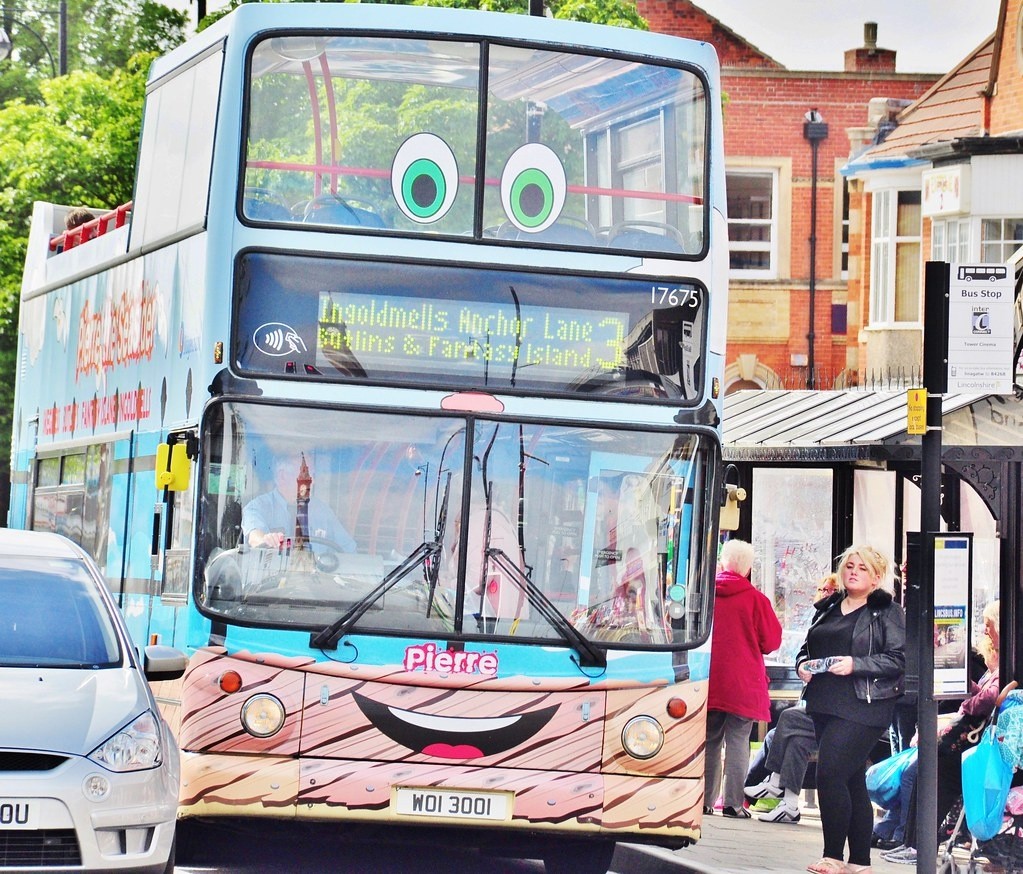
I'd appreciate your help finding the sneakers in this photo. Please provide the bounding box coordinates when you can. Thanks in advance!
[880,844,917,865]
[758,800,800,823]
[722,806,751,818]
[703,806,714,814]
[743,775,784,799]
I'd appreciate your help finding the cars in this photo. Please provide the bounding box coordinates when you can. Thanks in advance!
[32,494,116,552]
[0,527,184,873]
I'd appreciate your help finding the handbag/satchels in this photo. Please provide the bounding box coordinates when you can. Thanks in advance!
[961,725,1013,841]
[865,746,918,809]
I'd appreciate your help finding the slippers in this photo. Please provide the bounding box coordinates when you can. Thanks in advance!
[806,859,872,874]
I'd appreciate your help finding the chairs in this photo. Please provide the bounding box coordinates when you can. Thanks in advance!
[244,189,688,253]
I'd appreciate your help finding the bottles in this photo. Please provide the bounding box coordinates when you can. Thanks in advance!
[803,656,841,675]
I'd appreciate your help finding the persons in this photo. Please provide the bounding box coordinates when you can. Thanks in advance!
[705,539,782,819]
[57,208,99,254]
[241,447,358,583]
[713,574,893,826]
[871,600,999,864]
[796,543,906,874]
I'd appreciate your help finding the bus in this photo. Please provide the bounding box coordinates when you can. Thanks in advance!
[5,0,744,874]
[944,626,963,655]
